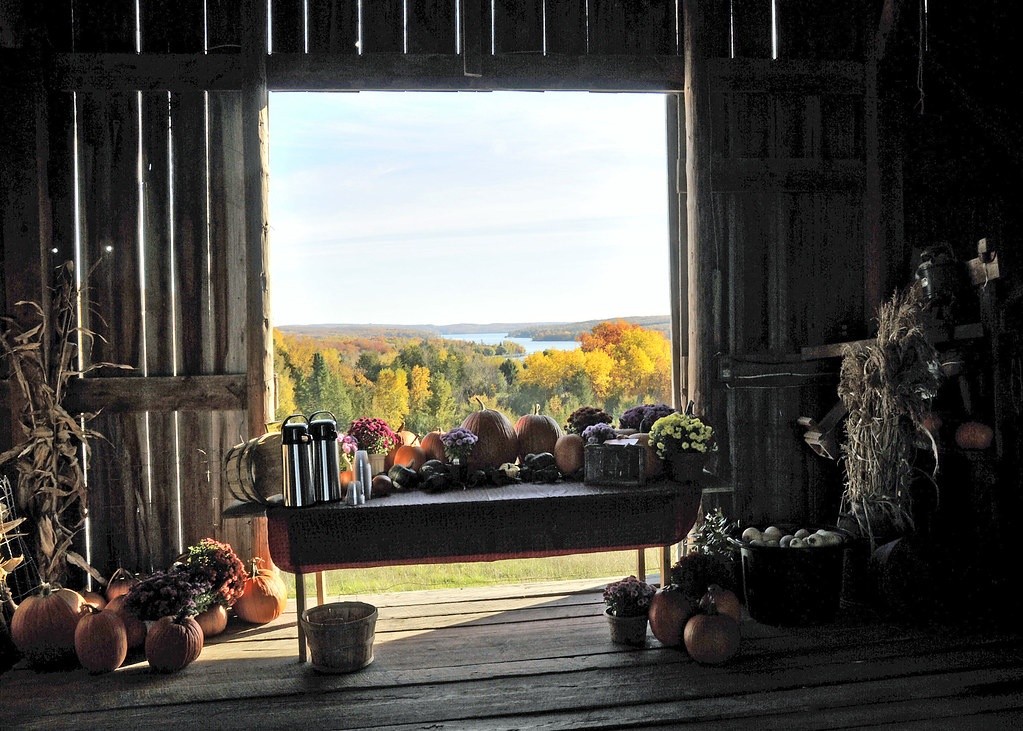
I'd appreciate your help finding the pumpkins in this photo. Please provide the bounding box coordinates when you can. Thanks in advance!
[384,397,585,474]
[10,558,287,672]
[647,572,745,668]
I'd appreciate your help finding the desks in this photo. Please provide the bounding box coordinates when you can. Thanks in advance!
[221,467,734,662]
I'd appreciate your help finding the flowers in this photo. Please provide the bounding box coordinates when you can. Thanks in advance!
[648,412,718,460]
[348,417,399,456]
[603,575,656,616]
[440,428,478,463]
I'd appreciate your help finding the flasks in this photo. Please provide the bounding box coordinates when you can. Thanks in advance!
[308,410,343,504]
[278,413,314,508]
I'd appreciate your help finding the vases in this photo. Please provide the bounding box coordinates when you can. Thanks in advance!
[671,453,705,482]
[369,454,386,475]
[603,606,647,643]
[444,462,468,482]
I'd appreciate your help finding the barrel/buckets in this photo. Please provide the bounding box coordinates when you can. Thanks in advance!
[724,521,860,629]
[299,601,378,675]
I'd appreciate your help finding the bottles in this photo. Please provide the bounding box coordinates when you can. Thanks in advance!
[353,448,371,500]
